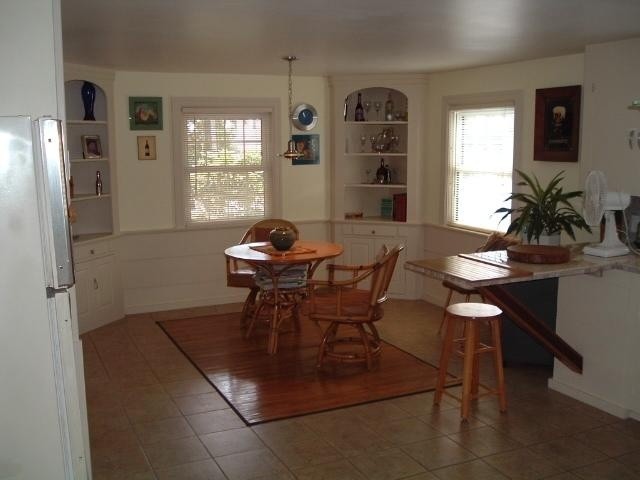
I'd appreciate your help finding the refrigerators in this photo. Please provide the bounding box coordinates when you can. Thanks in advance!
[0,114,91,480]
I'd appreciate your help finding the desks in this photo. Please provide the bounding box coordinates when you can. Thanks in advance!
[403,249,615,375]
[224,240,344,354]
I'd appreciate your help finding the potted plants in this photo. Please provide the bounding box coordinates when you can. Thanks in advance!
[489,168,599,246]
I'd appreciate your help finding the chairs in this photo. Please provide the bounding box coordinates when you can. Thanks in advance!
[307,240,405,370]
[226,217,299,329]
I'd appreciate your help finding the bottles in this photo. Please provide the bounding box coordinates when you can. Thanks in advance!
[95,170,103,197]
[385,100,394,121]
[144,139,150,156]
[376,157,391,184]
[355,92,364,121]
[81,80,96,120]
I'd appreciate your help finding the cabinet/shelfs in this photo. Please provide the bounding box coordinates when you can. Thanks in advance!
[64,79,115,244]
[72,238,126,337]
[342,87,408,225]
[340,224,424,301]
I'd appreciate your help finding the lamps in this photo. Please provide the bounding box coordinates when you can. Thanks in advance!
[276,56,305,160]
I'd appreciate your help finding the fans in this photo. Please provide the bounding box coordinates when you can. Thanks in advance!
[582,169,632,258]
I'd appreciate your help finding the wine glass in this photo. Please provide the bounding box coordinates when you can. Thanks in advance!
[358,132,400,153]
[363,101,371,121]
[374,101,381,121]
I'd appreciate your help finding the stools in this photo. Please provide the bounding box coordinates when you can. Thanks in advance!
[434,301,506,419]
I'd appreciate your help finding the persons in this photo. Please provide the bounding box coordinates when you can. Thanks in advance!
[296,140,314,160]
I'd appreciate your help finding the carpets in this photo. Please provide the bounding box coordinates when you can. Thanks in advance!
[155,312,463,426]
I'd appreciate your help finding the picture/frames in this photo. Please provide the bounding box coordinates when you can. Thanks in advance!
[81,135,102,159]
[137,135,156,160]
[129,96,163,130]
[291,134,320,164]
